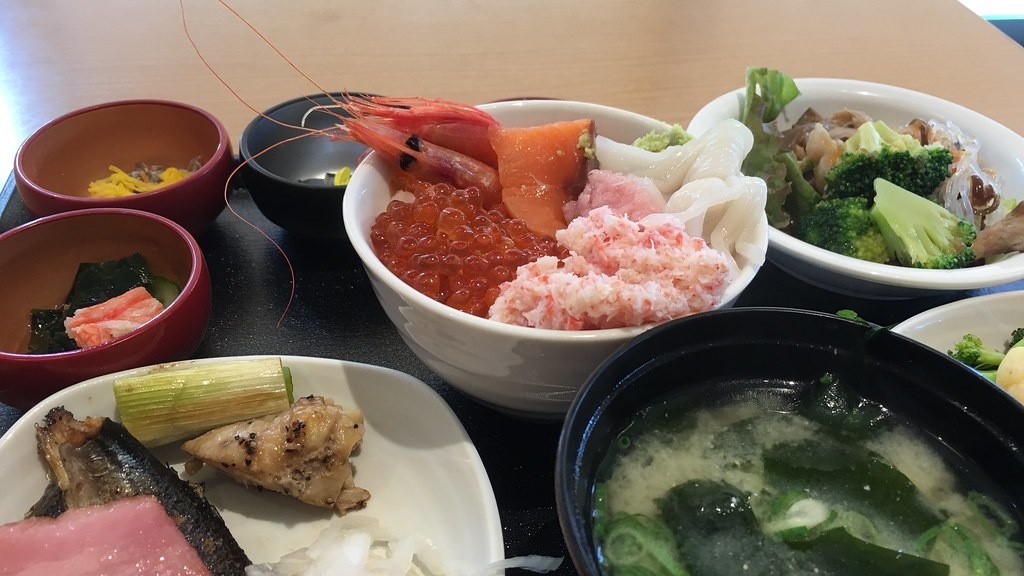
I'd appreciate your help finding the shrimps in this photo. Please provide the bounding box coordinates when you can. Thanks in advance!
[304,92,509,210]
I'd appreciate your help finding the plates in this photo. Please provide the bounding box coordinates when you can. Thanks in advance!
[2,355,509,574]
[890,288,1023,377]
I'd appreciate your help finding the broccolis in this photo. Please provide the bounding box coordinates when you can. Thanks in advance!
[944,326,1024,390]
[800,113,976,284]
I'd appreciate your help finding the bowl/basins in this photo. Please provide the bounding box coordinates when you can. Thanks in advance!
[240,91,405,239]
[342,100,764,413]
[554,305,1022,576]
[13,99,231,234]
[688,75,1023,298]
[0,209,215,415]
